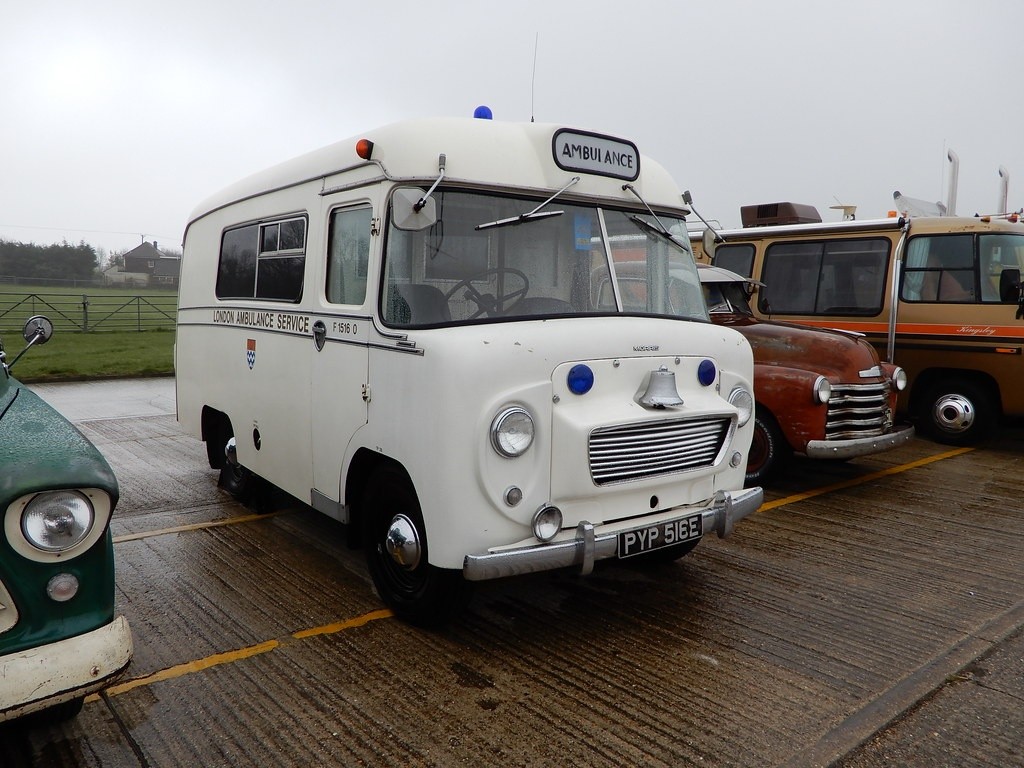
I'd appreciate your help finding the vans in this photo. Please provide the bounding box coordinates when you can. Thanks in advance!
[174,114,765,622]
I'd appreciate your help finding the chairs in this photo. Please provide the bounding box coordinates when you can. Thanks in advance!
[387,283,452,326]
[510,296,575,316]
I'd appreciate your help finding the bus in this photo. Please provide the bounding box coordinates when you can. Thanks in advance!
[581,212,1023,446]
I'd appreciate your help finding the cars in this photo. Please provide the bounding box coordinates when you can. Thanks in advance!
[0,313,137,735]
[574,255,916,484]
